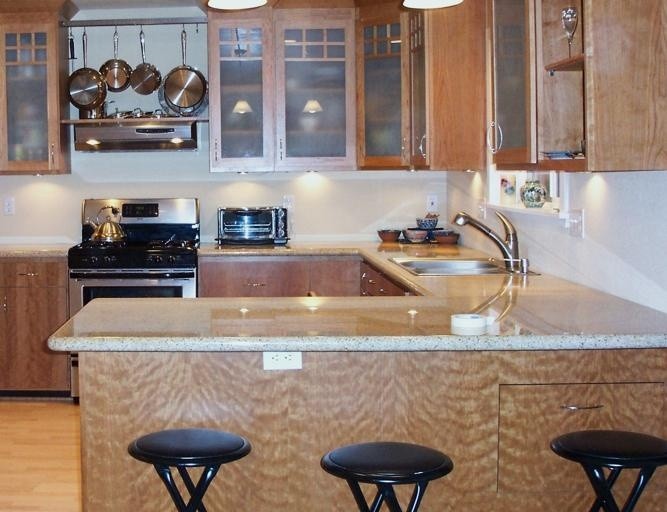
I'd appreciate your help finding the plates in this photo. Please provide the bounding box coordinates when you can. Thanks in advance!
[541,149,576,158]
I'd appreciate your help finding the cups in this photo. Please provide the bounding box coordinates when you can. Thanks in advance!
[26,145,35,161]
[37,147,43,160]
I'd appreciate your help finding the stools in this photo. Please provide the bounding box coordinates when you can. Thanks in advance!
[550,430,667,512]
[128,427,253,512]
[322,442,454,512]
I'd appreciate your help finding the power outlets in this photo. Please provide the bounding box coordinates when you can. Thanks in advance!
[263,352,302,371]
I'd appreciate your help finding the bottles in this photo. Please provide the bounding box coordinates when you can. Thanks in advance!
[13,144,24,160]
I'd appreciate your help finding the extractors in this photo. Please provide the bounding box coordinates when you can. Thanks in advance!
[74,122,197,150]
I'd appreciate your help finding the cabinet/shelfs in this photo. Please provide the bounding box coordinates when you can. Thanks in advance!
[198,256,310,298]
[410,1,493,171]
[0,0,81,176]
[311,257,362,297]
[361,262,407,296]
[0,257,70,398]
[357,1,413,170]
[273,8,359,170]
[487,0,535,165]
[535,0,666,171]
[209,5,275,172]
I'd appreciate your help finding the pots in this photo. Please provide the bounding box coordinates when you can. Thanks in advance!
[163,30,206,108]
[66,34,106,110]
[79,102,108,119]
[130,31,161,95]
[99,31,132,92]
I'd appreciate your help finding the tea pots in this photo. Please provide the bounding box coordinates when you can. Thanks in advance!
[89,206,126,241]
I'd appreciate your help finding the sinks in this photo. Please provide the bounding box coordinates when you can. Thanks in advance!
[388,257,540,276]
[453,210,519,272]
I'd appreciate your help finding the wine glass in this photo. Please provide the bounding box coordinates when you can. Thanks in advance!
[560,6,579,58]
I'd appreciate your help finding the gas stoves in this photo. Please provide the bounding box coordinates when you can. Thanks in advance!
[68,240,197,254]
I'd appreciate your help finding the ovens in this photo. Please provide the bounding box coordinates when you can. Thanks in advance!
[68,255,197,397]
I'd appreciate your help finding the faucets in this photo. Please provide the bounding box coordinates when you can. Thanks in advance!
[450,277,529,324]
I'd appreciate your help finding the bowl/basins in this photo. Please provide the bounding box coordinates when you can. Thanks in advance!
[298,117,321,132]
[402,230,428,244]
[435,234,460,244]
[377,229,402,242]
[415,218,438,229]
[432,230,454,240]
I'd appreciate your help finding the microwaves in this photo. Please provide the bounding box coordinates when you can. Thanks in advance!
[215,207,290,246]
